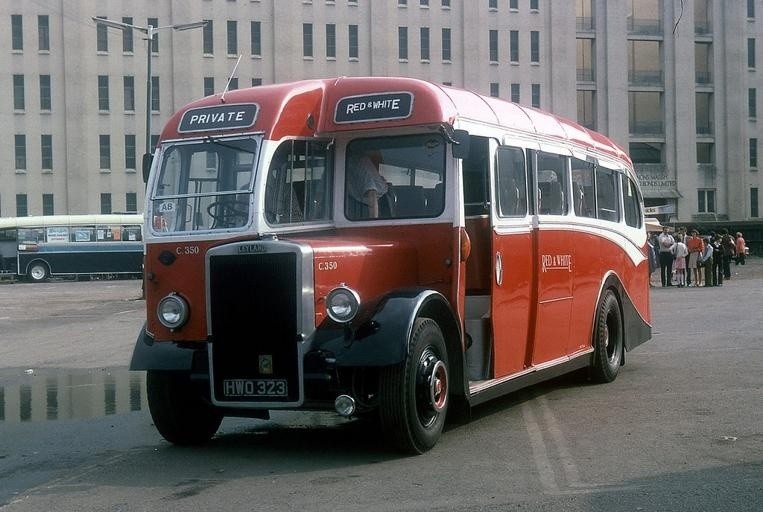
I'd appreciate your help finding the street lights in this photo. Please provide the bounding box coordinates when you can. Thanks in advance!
[91,16,208,153]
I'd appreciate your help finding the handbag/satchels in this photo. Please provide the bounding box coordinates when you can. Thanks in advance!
[671,254,677,260]
[696,251,705,268]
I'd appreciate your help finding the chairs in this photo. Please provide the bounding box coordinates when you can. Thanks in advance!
[287,171,584,219]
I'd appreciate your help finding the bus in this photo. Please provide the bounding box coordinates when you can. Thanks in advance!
[130,76,652,454]
[0,214,144,284]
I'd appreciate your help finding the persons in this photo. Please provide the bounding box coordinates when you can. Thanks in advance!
[646,227,747,287]
[313,147,393,220]
[233,168,304,226]
